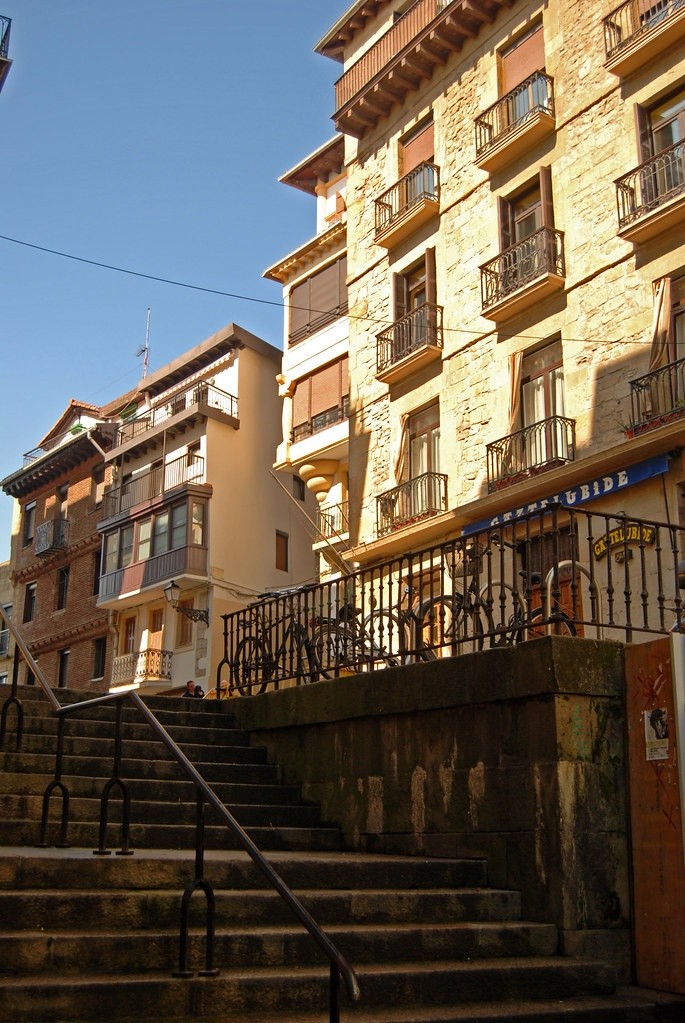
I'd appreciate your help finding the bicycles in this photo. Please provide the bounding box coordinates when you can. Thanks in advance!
[234,582,433,697]
[415,530,576,662]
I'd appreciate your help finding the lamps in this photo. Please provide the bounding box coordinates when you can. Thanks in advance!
[163,578,209,628]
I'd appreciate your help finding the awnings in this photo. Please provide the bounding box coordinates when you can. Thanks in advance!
[460,451,669,537]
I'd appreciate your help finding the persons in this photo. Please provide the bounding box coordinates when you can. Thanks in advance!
[195,686,204,698]
[182,680,199,697]
